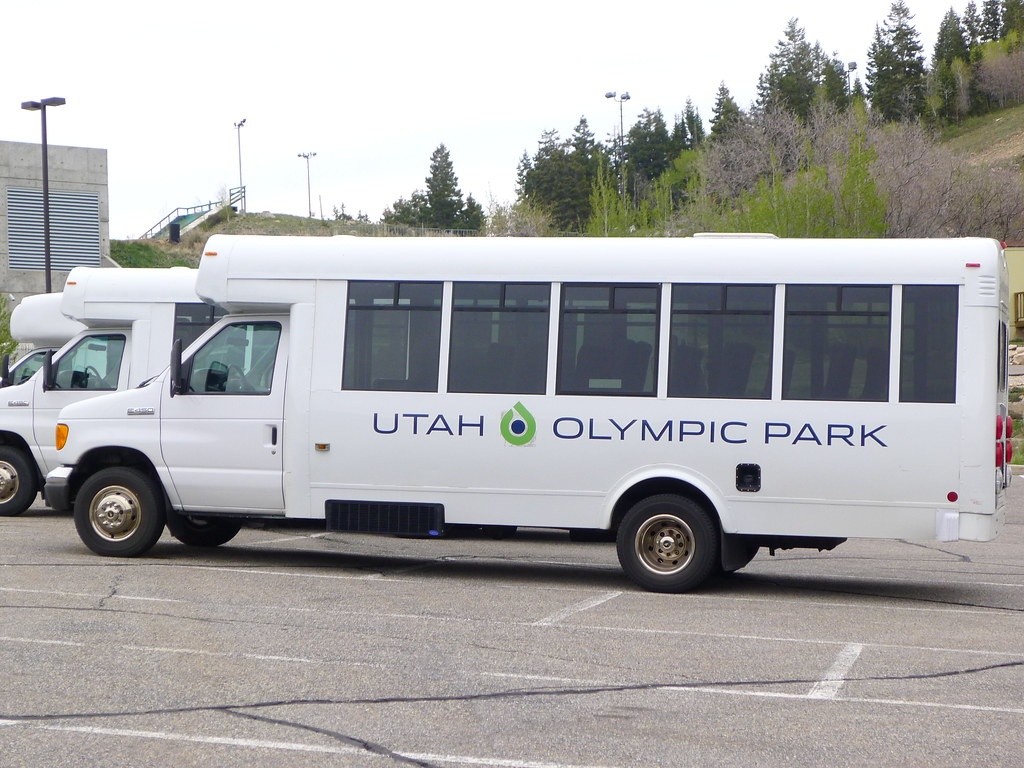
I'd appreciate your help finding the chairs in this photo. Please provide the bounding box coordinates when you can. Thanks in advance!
[822,344,856,397]
[718,342,756,395]
[668,344,705,396]
[572,340,653,392]
[858,346,888,398]
[479,342,514,389]
[760,349,796,399]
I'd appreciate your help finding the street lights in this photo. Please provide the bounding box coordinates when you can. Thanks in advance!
[605,90,630,210]
[20,97,66,293]
[234,118,247,209]
[298,152,317,217]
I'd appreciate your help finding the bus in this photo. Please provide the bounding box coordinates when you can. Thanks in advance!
[0,234,1018,595]
